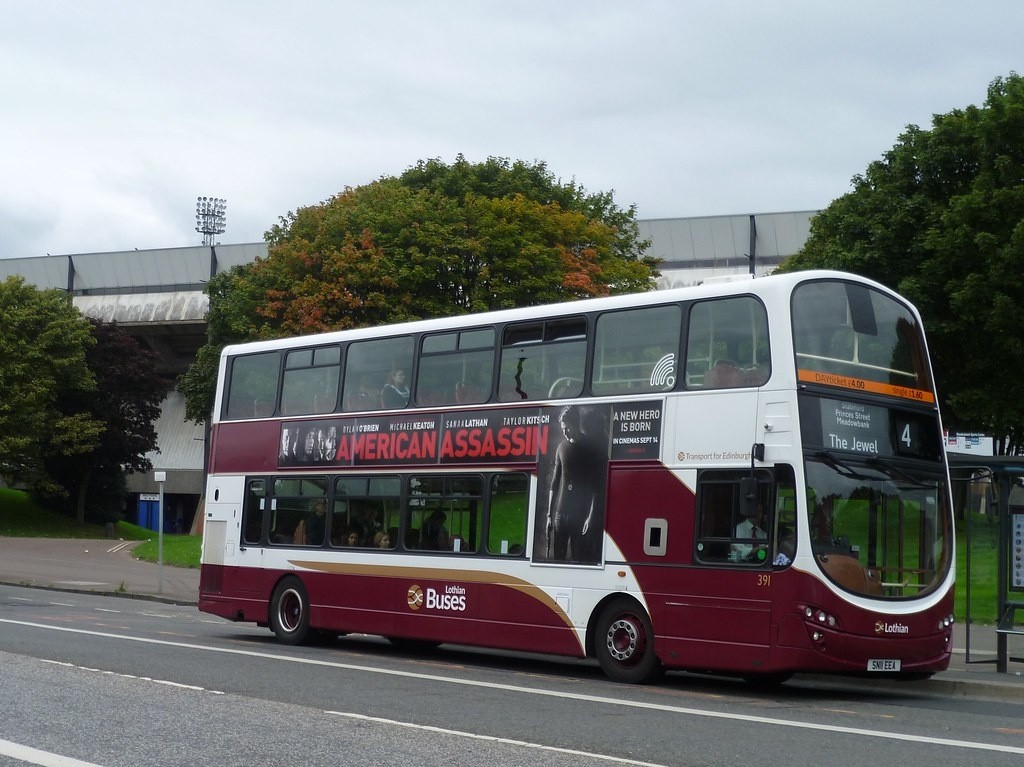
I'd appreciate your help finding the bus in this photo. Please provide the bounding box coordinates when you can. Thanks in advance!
[199,271,1001,682]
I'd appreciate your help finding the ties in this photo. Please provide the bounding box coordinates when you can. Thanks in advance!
[752,528,759,548]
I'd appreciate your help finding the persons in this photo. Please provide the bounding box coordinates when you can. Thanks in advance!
[420,511,466,551]
[545,407,604,561]
[305,500,326,546]
[348,528,360,546]
[509,544,523,554]
[733,505,768,557]
[373,531,392,548]
[381,369,411,409]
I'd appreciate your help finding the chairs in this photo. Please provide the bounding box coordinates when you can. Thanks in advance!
[244,496,469,553]
[229,360,769,419]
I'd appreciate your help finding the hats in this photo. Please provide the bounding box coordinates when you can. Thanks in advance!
[374,532,387,546]
